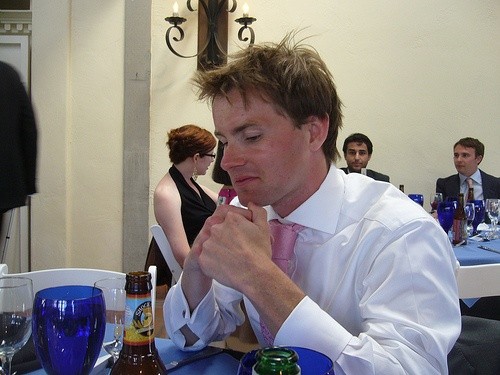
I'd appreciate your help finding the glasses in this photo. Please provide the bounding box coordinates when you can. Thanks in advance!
[453,152,482,158]
[199,153,216,158]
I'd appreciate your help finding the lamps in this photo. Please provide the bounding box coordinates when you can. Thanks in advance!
[164,0,257,71]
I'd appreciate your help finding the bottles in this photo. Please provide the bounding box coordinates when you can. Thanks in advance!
[108,270,170,375]
[467,188,476,202]
[452,193,468,245]
[251,346,302,375]
[400,184,404,193]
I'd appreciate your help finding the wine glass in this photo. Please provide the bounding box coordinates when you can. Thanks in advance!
[429,192,500,245]
[93,277,128,363]
[0,276,35,374]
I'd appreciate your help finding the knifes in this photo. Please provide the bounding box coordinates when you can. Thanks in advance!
[164,346,223,370]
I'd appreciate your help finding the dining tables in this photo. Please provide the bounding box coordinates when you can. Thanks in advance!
[23,336,239,375]
[453,221,500,306]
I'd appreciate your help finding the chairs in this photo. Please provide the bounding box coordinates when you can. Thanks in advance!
[0,264,130,341]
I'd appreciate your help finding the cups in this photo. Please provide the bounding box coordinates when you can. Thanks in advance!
[408,194,424,207]
[31,285,108,375]
[235,346,336,375]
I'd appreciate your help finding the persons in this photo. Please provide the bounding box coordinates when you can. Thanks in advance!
[154,125,238,270]
[163,26,462,375]
[435,138,500,202]
[340,133,390,183]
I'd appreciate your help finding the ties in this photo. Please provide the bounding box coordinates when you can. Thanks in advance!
[259,219,306,347]
[466,178,474,203]
